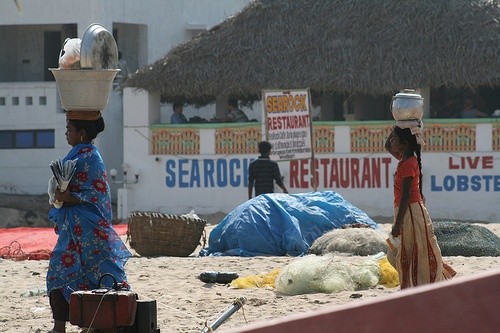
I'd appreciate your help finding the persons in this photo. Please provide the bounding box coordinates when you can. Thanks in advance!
[217,100,250,122]
[385,127,457,289]
[430,96,500,119]
[171,101,189,124]
[47,111,132,333]
[248,142,288,200]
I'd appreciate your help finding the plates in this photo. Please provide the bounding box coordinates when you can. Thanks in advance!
[79,23,118,70]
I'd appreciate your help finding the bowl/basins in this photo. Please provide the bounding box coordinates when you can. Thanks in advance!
[48,68,121,112]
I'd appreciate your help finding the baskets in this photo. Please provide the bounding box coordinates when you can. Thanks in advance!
[127,212,207,257]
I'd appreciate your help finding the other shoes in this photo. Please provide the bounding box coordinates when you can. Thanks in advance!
[48,329,66,333]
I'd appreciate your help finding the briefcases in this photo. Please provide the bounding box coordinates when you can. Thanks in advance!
[69,273,138,327]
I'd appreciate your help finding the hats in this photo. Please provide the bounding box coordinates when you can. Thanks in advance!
[66,111,101,120]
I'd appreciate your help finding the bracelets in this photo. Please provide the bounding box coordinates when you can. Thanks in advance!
[79,200,82,204]
